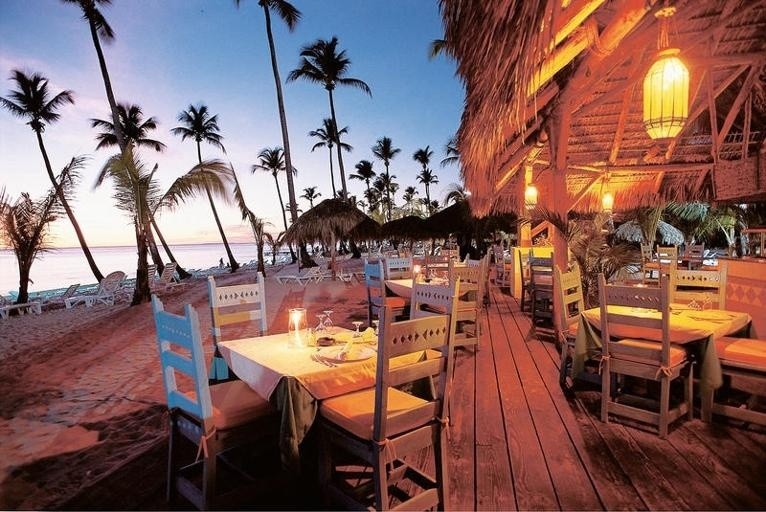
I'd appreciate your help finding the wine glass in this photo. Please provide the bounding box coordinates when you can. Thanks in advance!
[316,314,326,338]
[372,320,379,343]
[323,310,334,334]
[429,269,435,278]
[433,267,437,277]
[352,322,364,344]
[442,271,448,281]
[416,273,427,284]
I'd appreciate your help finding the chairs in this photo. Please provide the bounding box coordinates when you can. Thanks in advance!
[316,306,455,511]
[405,273,462,424]
[263,245,430,289]
[205,270,269,382]
[0,257,259,320]
[149,293,269,511]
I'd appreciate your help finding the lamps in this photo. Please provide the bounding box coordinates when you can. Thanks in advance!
[600,173,613,213]
[522,163,539,214]
[640,1,691,146]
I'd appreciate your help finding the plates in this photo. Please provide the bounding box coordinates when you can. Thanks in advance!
[319,342,376,362]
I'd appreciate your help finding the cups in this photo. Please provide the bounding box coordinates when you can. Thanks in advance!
[305,329,316,346]
